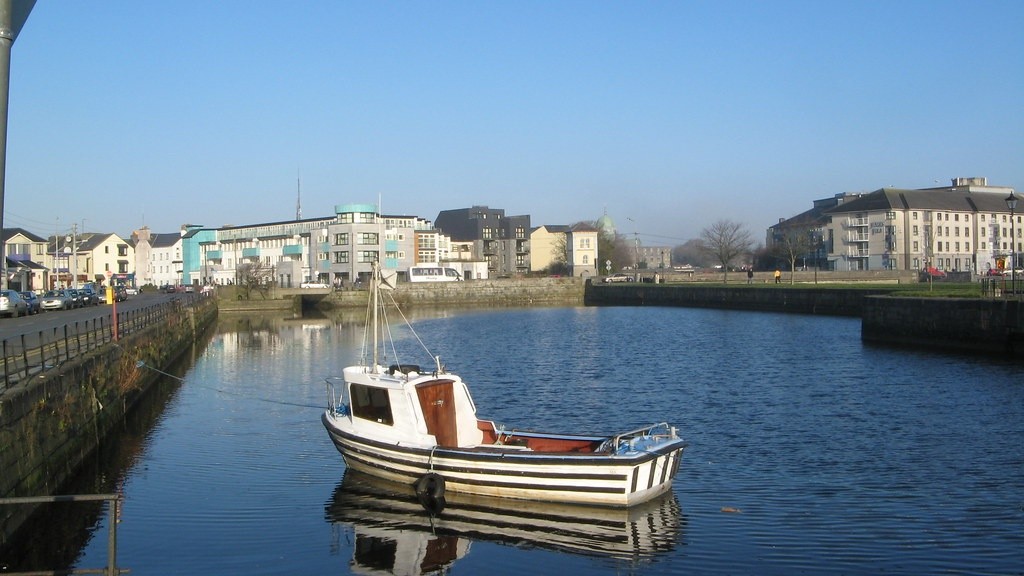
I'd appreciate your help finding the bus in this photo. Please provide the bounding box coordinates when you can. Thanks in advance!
[406,266,465,283]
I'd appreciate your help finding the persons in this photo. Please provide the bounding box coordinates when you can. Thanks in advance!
[774,269,781,284]
[333,277,361,289]
[748,268,753,284]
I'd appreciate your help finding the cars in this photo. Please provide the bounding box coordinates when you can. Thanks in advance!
[0,289,30,319]
[1001,268,1024,277]
[16,290,41,315]
[180,284,194,293]
[119,286,127,302]
[299,280,332,289]
[96,287,122,303]
[125,287,138,295]
[76,288,100,307]
[162,285,175,293]
[921,266,944,282]
[39,289,74,311]
[602,273,634,283]
[987,269,1000,276]
[66,288,80,308]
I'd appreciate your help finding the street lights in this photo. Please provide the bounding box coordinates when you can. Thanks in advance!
[1004,190,1019,297]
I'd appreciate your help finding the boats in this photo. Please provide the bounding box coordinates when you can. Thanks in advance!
[319,261,690,510]
[322,469,684,576]
